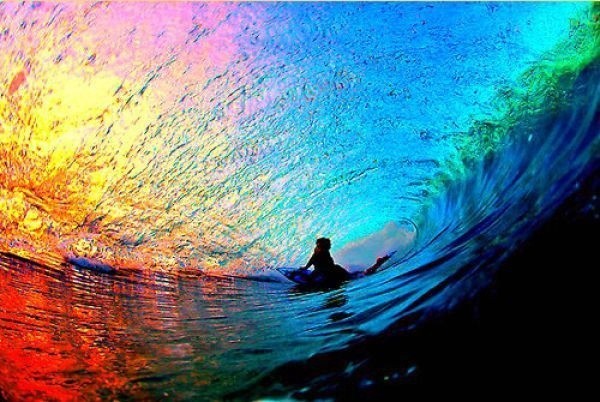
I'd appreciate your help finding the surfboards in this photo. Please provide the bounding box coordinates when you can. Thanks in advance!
[277,268,313,284]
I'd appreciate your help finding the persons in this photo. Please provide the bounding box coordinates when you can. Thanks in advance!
[298,238,341,292]
